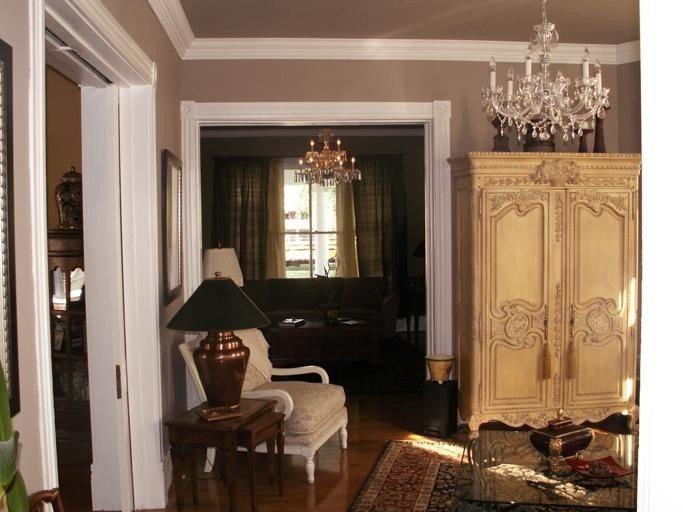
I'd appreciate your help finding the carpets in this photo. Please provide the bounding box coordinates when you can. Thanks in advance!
[348,440,597,511]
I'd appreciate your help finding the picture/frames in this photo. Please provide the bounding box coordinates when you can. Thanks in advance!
[161,149,184,305]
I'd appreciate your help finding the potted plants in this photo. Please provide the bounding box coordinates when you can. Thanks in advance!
[316,266,340,324]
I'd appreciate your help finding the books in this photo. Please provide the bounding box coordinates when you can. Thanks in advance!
[279,318,305,327]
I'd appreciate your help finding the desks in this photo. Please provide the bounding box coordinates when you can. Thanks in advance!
[164,396,287,512]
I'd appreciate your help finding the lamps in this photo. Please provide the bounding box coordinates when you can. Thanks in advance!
[166,269,270,422]
[478,0,611,146]
[204,242,246,287]
[290,126,361,188]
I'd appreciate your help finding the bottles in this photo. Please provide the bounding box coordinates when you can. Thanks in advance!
[53,164,82,229]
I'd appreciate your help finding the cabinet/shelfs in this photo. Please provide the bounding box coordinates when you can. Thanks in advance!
[46,226,94,467]
[445,151,637,440]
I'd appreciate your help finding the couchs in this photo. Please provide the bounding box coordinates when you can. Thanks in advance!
[239,278,399,328]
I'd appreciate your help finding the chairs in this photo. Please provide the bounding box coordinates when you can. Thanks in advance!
[177,325,347,483]
